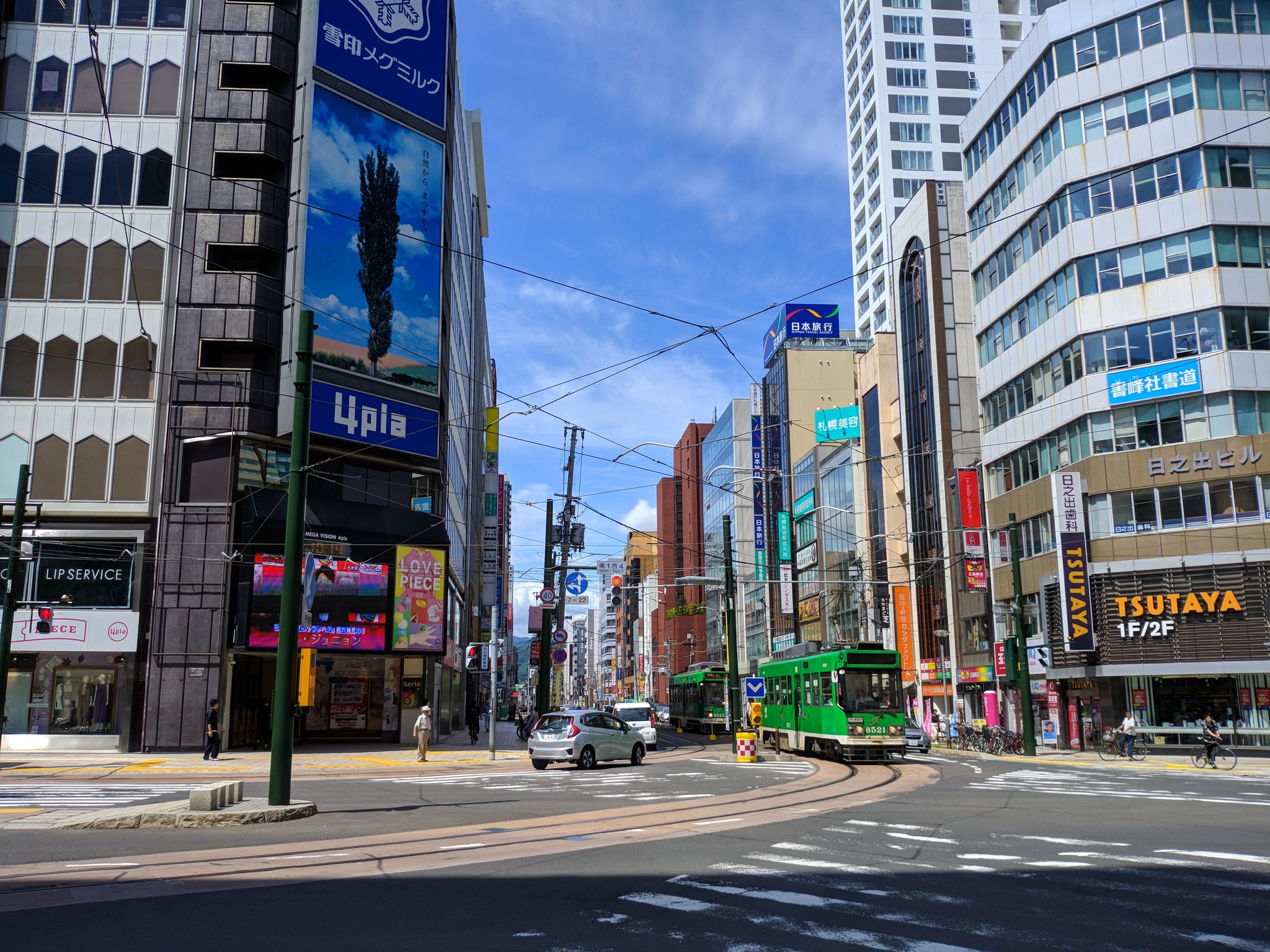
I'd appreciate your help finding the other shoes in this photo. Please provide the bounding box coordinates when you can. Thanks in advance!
[1119,754,1125,757]
[486,729,489,732]
[202,757,209,760]
[475,736,479,741]
[418,760,428,762]
[209,757,220,761]
[1127,759,1133,761]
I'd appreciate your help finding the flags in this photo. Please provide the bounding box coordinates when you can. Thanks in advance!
[906,696,931,737]
[944,701,959,738]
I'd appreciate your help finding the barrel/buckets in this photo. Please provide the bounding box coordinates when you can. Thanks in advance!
[736,732,757,763]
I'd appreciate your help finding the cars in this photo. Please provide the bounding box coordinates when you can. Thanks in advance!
[528,710,646,770]
[905,716,931,754]
[559,699,670,723]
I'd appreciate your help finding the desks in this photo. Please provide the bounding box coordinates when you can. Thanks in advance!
[116,17,148,27]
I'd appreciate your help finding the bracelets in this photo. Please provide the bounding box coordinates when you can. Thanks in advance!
[1218,737,1219,739]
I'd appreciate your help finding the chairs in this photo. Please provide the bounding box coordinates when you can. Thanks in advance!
[157,6,187,29]
[587,715,600,728]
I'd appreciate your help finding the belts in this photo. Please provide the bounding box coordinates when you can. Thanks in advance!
[420,728,429,730]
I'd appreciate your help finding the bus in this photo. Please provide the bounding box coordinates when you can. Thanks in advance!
[669,662,733,735]
[757,589,921,762]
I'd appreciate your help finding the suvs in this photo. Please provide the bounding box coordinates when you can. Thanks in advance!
[611,702,657,750]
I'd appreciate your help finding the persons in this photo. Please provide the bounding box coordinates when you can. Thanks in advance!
[764,693,788,705]
[931,709,939,744]
[1116,710,1136,761]
[77,675,93,734]
[710,691,722,702]
[1202,712,1223,768]
[202,698,225,760]
[517,705,533,716]
[855,688,874,710]
[465,699,483,741]
[420,699,433,748]
[413,706,431,762]
[746,698,756,728]
[59,699,78,730]
[93,674,109,734]
[482,700,490,732]
[1043,721,1055,739]
[253,699,272,750]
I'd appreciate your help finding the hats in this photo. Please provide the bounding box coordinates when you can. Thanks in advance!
[421,705,431,711]
[485,700,491,704]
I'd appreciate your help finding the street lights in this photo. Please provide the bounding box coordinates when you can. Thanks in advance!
[583,686,590,708]
[932,630,951,749]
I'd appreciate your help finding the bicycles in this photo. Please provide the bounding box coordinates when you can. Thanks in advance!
[516,710,533,741]
[951,720,1024,756]
[1191,736,1237,771]
[1098,727,1151,761]
[467,718,482,745]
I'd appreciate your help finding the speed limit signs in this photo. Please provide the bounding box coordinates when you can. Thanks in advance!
[540,588,555,603]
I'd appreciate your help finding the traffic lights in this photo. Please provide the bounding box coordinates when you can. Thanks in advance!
[650,691,653,697]
[37,606,54,633]
[530,669,535,678]
[611,574,622,606]
[751,703,762,726]
[666,688,669,694]
[489,639,504,657]
[521,690,524,696]
[1037,647,1049,667]
[466,646,479,670]
[570,695,572,700]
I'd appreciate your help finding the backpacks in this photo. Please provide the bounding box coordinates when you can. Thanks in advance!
[469,706,479,720]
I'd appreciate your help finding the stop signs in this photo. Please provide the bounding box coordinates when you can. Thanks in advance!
[641,688,645,692]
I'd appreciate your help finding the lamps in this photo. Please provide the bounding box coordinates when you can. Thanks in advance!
[1169,273,1177,277]
[1116,345,1140,350]
[111,653,126,665]
[1099,206,1119,210]
[1083,50,1109,55]
[1159,676,1162,683]
[1086,677,1089,684]
[1214,674,1219,680]
[34,653,41,663]
[1056,678,1061,686]
[1207,17,1237,24]
[1229,162,1249,168]
[1176,347,1188,351]
[1144,329,1178,338]
[1129,177,1155,187]
[1154,676,1159,682]
[1105,272,1119,276]
[1204,675,1208,682]
[61,593,74,602]
[13,651,18,662]
